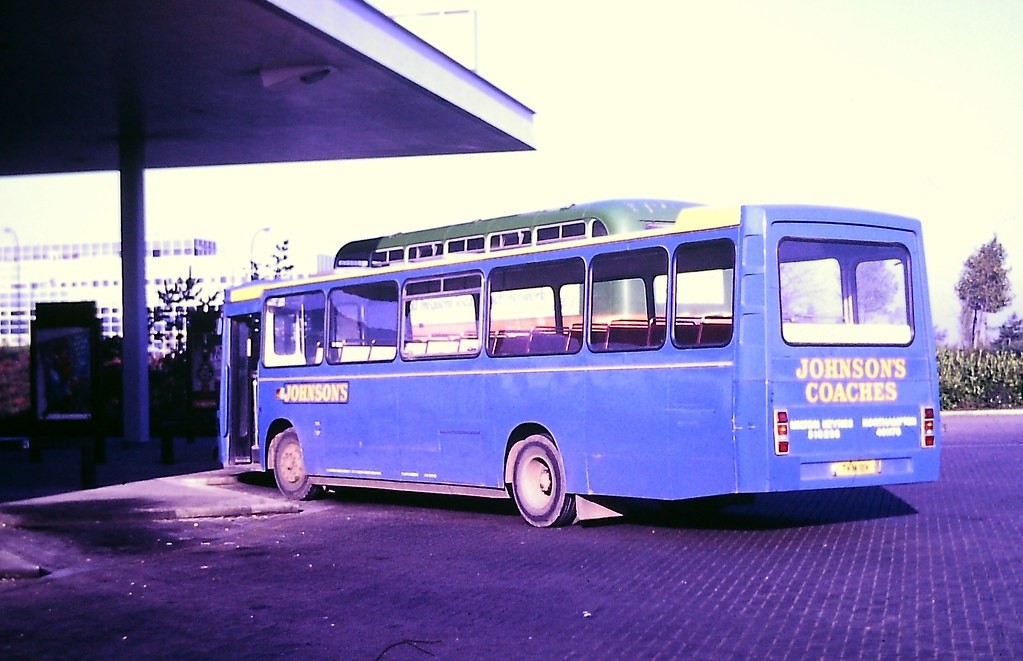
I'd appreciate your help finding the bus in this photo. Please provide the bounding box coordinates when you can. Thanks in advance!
[333,197,733,335]
[213,205,942,530]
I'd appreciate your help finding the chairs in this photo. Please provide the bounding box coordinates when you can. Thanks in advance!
[308,311,739,372]
